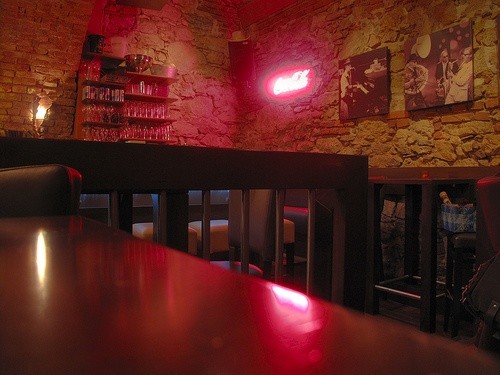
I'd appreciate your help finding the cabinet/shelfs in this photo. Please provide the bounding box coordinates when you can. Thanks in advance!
[71,50,178,146]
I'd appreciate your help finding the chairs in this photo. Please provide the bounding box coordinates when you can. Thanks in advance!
[463,175,500,354]
[0,164,83,218]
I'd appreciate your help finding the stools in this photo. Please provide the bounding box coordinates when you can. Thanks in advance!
[443,232,477,338]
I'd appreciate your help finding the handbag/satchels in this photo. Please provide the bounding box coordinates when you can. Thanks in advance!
[457,251,500,352]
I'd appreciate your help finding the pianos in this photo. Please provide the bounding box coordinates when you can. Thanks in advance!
[353,68,387,115]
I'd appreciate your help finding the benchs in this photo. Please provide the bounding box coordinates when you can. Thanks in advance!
[132,219,296,291]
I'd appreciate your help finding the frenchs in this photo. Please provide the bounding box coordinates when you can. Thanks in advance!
[403,35,431,95]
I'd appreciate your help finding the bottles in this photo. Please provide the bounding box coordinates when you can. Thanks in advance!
[439,191,452,204]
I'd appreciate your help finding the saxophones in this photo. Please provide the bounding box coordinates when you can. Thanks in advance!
[442,57,464,97]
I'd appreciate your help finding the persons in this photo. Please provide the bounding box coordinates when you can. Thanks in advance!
[404,45,473,109]
[341,55,387,117]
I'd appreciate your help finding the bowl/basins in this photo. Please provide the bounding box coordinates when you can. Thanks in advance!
[150,63,178,77]
[124,53,153,73]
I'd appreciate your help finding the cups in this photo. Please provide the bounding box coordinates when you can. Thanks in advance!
[82,104,172,141]
